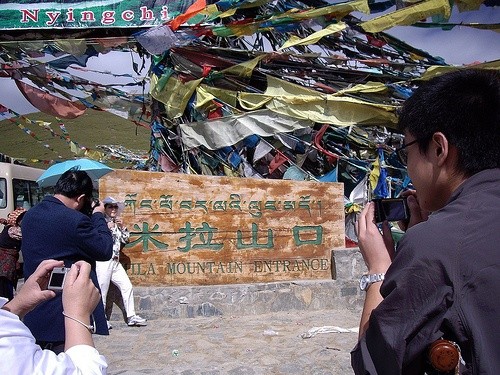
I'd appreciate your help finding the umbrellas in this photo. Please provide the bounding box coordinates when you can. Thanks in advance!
[36,158,114,188]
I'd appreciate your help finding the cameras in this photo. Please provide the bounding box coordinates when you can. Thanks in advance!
[47,267,79,293]
[369,198,409,224]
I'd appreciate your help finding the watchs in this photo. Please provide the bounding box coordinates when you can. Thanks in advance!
[359,274,385,291]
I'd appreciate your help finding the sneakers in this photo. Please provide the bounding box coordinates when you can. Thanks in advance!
[127,315,147,326]
[106,321,113,330]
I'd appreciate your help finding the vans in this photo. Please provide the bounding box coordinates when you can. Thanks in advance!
[0,160,48,226]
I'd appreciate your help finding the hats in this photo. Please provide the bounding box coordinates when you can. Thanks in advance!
[103,197,121,204]
[8,207,28,227]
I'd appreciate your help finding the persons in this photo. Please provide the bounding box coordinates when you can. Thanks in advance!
[0,206,29,301]
[21,165,113,355]
[350,68,500,375]
[0,259,108,375]
[96,197,148,330]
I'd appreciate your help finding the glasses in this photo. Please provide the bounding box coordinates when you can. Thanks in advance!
[109,205,118,209]
[385,139,420,169]
